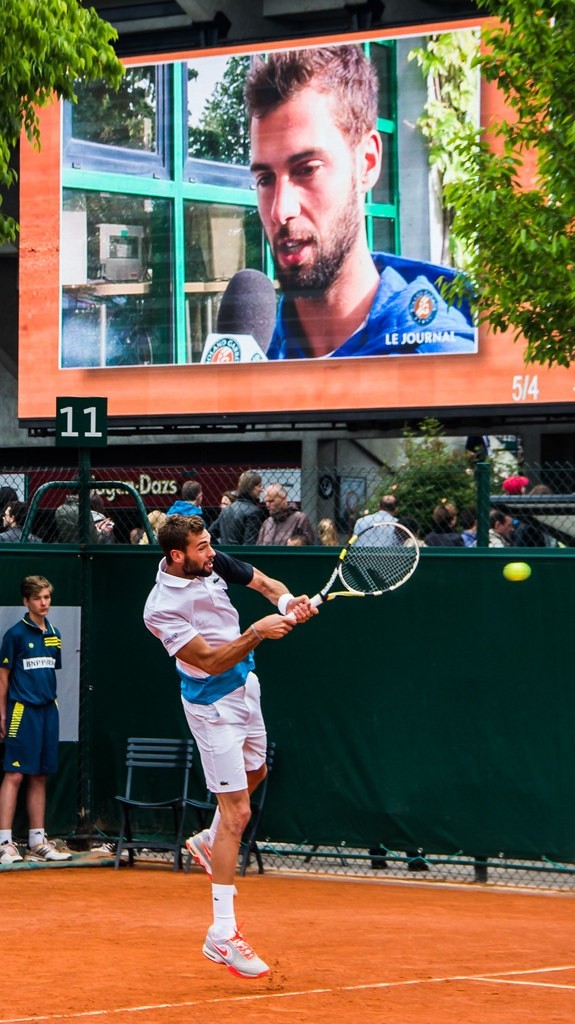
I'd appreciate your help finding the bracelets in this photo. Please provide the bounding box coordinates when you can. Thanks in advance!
[251,625,263,641]
[278,594,294,615]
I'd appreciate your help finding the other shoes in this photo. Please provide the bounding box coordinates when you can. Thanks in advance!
[90,842,129,856]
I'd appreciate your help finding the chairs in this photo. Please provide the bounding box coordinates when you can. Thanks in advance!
[114,736,276,878]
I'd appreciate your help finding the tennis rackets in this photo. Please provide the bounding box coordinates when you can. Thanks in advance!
[284,523,420,620]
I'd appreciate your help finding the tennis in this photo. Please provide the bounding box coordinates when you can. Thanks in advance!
[503,562,532,581]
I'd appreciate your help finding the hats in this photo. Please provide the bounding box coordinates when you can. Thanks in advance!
[503,476,528,494]
[224,490,237,504]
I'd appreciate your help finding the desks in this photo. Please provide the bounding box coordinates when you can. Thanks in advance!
[60,279,281,366]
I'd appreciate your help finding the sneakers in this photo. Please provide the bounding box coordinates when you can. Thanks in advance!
[202,925,269,979]
[23,837,73,862]
[0,840,23,864]
[185,829,238,897]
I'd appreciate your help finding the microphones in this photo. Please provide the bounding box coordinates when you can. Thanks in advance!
[200,269,276,364]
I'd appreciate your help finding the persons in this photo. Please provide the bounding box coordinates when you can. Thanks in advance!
[144,513,317,979]
[91,842,117,854]
[0,576,73,864]
[368,848,429,872]
[242,44,477,361]
[0,476,556,549]
